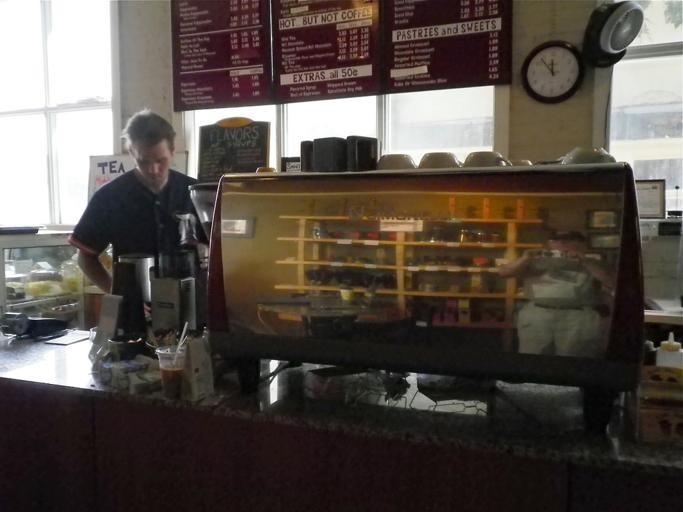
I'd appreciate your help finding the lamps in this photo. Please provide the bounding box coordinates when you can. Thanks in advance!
[584,1,644,67]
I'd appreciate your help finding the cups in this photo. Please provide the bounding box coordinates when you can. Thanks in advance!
[101,334,143,362]
[378,149,535,173]
[154,343,188,400]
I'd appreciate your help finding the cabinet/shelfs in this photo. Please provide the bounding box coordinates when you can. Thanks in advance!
[0,230,85,332]
[207,160,645,445]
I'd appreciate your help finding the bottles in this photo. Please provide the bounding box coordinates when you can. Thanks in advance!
[657,329,683,371]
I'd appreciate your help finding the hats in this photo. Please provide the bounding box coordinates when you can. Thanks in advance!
[556,229,587,241]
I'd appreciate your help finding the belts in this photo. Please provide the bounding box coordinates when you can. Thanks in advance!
[535,303,584,310]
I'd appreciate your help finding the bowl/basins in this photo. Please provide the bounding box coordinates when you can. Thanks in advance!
[560,144,616,165]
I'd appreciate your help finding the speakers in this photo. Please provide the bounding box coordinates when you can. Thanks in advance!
[300,140,313,172]
[346,136,378,172]
[312,137,346,172]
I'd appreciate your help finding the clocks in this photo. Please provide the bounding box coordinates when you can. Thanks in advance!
[521,40,583,104]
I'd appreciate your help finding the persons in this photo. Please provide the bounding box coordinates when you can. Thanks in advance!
[66,109,212,317]
[497,230,612,358]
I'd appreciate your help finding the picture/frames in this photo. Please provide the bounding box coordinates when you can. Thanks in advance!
[635,179,667,219]
